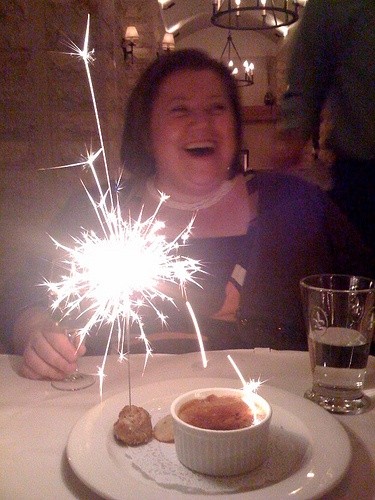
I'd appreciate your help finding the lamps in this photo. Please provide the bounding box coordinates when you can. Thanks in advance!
[157,33,176,58]
[220,12,255,87]
[211,0,300,31]
[121,25,139,64]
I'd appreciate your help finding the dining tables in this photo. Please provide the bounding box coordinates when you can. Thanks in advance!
[0,348,375,500]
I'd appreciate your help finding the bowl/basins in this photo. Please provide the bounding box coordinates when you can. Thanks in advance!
[170,387,272,477]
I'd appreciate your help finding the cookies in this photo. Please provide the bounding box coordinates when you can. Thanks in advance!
[153,415,174,443]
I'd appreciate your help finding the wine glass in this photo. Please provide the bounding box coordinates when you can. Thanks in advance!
[48,256,96,392]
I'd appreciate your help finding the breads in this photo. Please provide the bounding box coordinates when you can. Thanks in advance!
[112,405,153,446]
[179,395,266,430]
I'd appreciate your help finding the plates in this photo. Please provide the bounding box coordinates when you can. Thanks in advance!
[63,376,351,500]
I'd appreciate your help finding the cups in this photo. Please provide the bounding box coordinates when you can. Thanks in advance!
[298,273,375,415]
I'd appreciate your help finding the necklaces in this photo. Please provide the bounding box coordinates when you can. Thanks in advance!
[146,175,239,210]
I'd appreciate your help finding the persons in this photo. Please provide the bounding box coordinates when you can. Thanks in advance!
[0,48,375,381]
[270,0,375,251]
